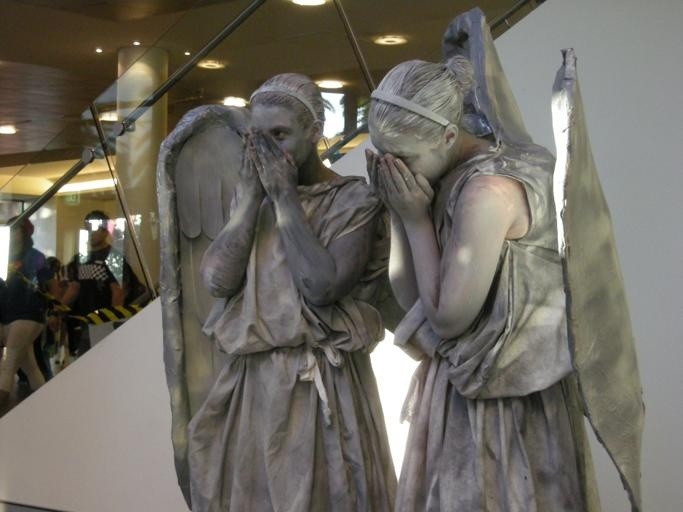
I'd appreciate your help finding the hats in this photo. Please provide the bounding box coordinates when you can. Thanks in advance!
[5,215,34,237]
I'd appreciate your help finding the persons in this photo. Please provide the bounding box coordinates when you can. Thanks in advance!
[361,54,599,512]
[0,208,140,409]
[186,72,399,512]
